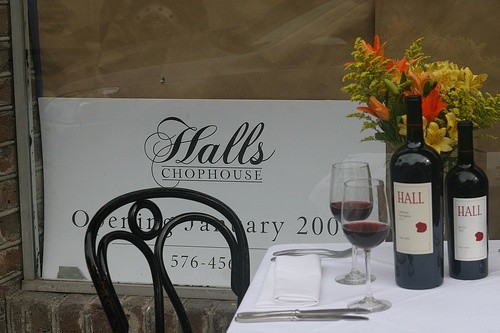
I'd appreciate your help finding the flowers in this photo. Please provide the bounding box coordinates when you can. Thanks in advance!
[340,34,500,174]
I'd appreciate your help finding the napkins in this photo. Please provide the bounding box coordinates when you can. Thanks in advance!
[256,253,322,308]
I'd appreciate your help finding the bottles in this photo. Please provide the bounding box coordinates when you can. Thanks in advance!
[389,97,445,289]
[447,119,488,280]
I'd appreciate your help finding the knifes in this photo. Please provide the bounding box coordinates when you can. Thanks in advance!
[237,308,371,315]
[234,315,369,321]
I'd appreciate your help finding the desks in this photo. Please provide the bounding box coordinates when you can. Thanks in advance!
[226,239,500,333]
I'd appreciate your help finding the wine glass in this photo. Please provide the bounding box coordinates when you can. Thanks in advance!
[329,162,377,284]
[342,179,392,313]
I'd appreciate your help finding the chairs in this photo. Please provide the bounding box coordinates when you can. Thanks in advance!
[85,187,250,333]
